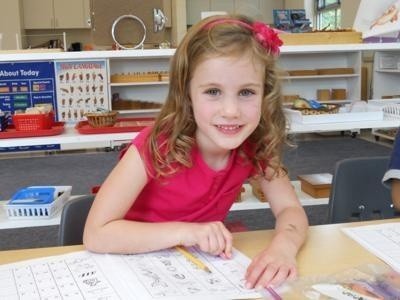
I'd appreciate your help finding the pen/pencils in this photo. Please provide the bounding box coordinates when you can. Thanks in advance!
[264,287,282,300]
[175,245,211,272]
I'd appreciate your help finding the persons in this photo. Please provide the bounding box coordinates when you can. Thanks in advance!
[81,14,311,292]
[382,126,400,214]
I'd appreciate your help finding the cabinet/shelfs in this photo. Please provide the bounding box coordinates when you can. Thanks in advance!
[22,0,93,31]
[0,40,363,147]
[363,42,400,147]
[0,1,29,51]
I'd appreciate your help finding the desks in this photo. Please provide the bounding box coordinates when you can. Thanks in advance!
[0,215,400,300]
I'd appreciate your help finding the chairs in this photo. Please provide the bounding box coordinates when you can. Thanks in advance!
[57,192,96,246]
[326,155,395,224]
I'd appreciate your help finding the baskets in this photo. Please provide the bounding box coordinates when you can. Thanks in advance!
[367,99,400,118]
[2,185,73,220]
[84,111,120,128]
[13,111,54,133]
